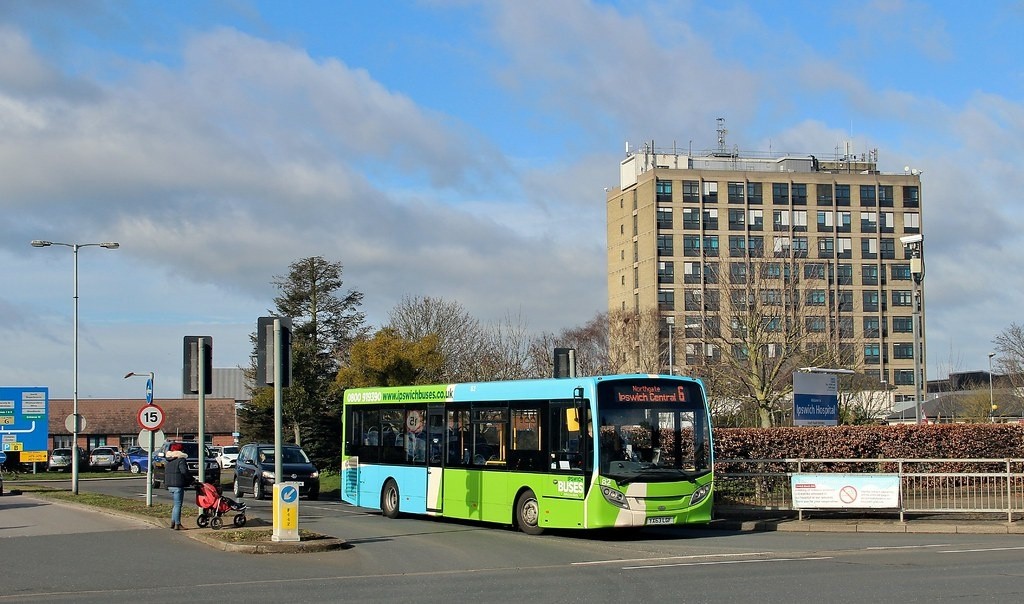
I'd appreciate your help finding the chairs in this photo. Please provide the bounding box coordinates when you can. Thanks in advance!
[367,425,494,465]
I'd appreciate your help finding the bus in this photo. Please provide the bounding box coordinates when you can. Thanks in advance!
[341,373,715,536]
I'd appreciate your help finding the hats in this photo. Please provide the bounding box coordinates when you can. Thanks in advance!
[171,444,182,451]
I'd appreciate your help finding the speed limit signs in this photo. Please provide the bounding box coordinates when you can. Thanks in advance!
[137,404,165,431]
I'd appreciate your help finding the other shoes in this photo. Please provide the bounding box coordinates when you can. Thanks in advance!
[237,505,246,510]
[238,502,245,505]
[170,522,175,528]
[174,524,188,530]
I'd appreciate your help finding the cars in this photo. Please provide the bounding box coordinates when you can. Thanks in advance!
[87,448,118,471]
[49,446,88,473]
[123,446,169,474]
[97,446,122,467]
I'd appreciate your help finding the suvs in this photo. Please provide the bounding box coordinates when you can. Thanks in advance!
[232,441,321,500]
[152,440,221,490]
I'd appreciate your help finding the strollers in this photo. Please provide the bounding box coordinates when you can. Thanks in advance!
[191,478,247,530]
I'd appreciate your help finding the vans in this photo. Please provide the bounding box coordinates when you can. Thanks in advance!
[207,445,240,469]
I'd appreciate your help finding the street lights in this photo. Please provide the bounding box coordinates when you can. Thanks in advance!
[123,371,154,506]
[30,240,120,494]
[988,352,997,424]
[880,380,887,420]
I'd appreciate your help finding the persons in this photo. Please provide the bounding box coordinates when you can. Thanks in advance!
[212,483,246,511]
[164,444,196,530]
